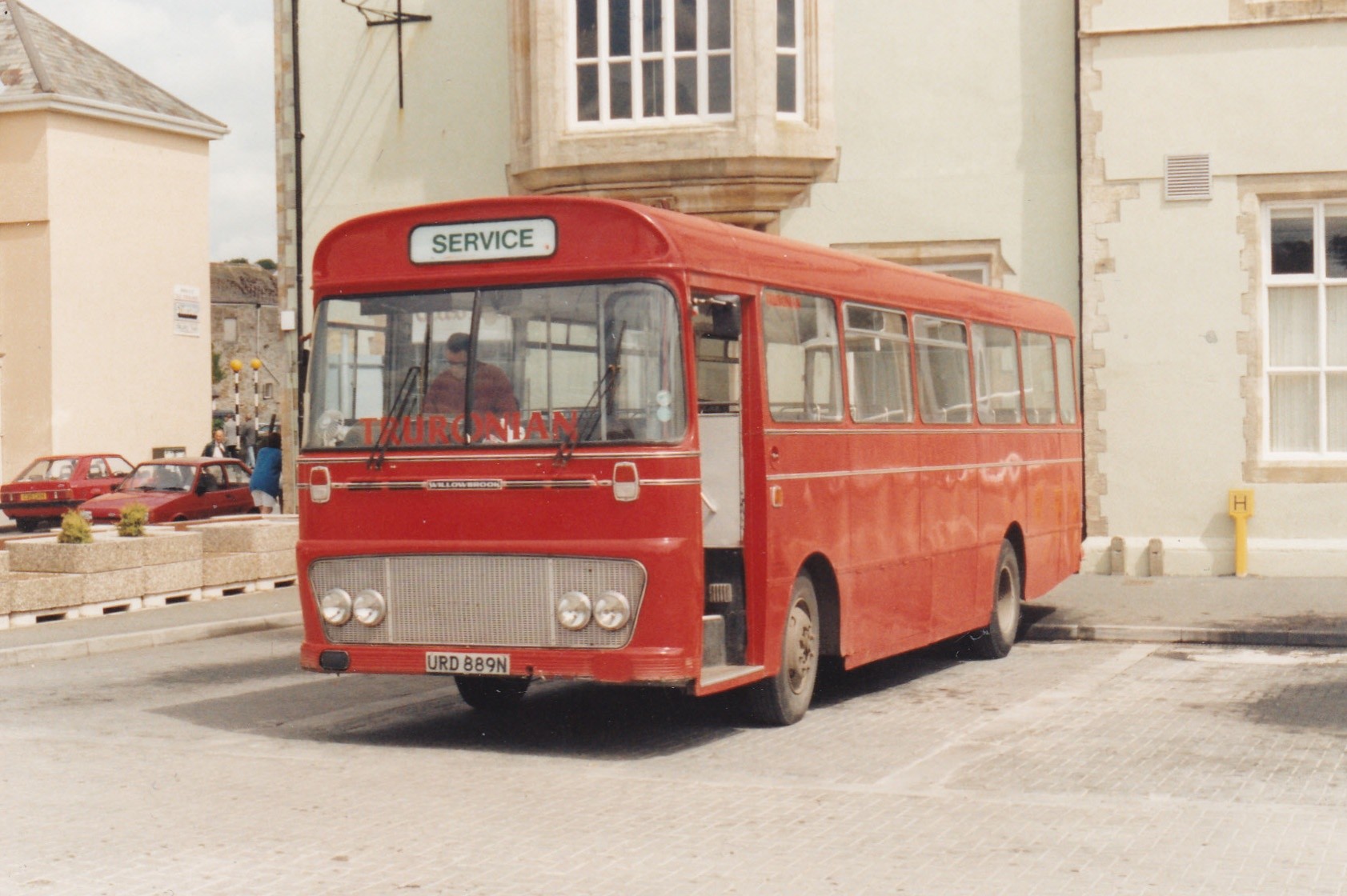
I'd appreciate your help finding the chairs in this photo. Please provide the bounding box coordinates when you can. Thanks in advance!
[88,464,102,479]
[154,473,180,488]
[780,403,1072,424]
[184,473,219,492]
[58,465,72,479]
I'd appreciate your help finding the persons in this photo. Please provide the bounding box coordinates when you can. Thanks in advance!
[250,432,283,514]
[424,333,519,413]
[201,423,233,485]
[239,415,256,469]
[222,411,237,458]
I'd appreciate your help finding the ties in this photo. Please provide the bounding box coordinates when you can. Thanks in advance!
[218,445,226,457]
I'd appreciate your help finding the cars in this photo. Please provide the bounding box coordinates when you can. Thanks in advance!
[0,454,153,534]
[77,457,260,526]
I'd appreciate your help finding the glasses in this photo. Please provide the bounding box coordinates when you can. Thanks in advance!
[447,360,469,367]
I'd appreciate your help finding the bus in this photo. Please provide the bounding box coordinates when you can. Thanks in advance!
[296,196,1085,729]
